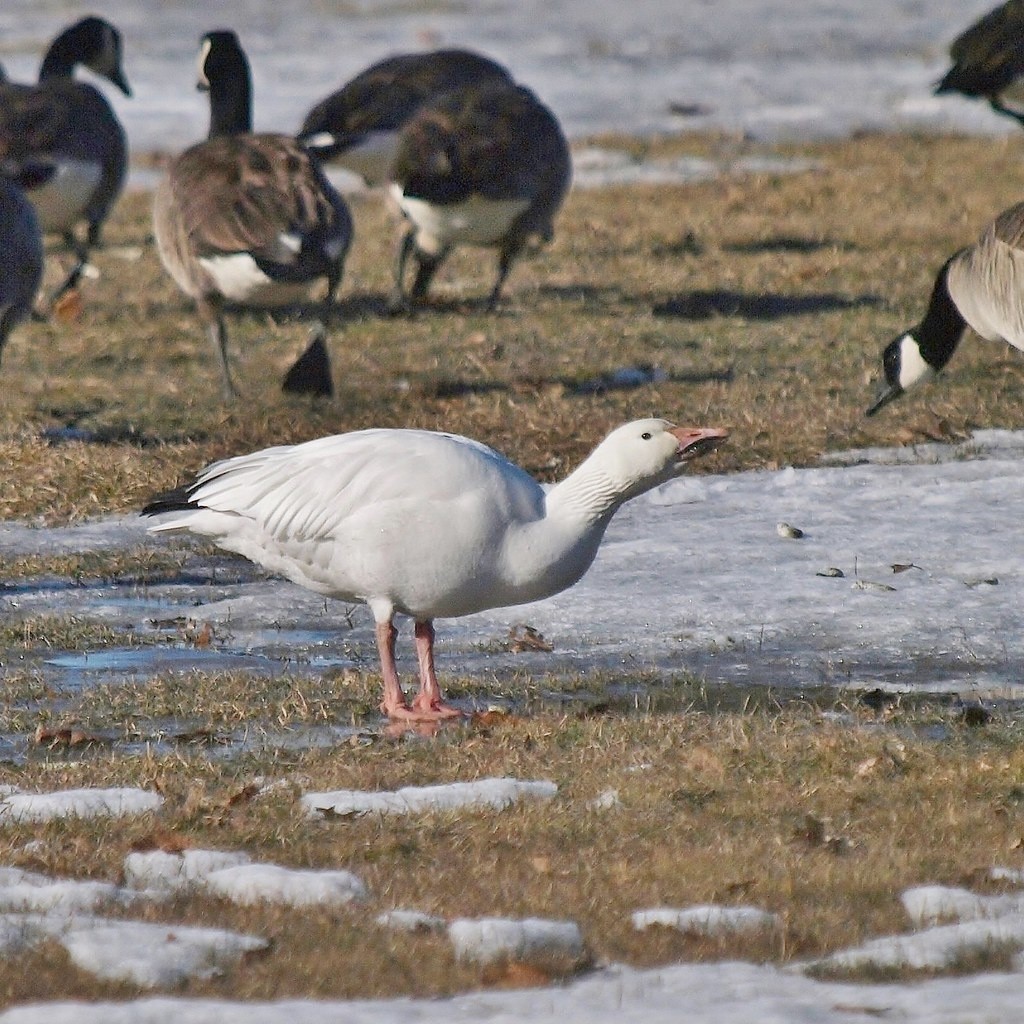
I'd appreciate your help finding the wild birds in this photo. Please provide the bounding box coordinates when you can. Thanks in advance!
[37,14,133,256]
[150,28,353,407]
[383,80,570,322]
[863,194,1024,423]
[138,417,734,725]
[294,48,557,316]
[0,58,104,321]
[0,161,45,352]
[931,0,1023,128]
